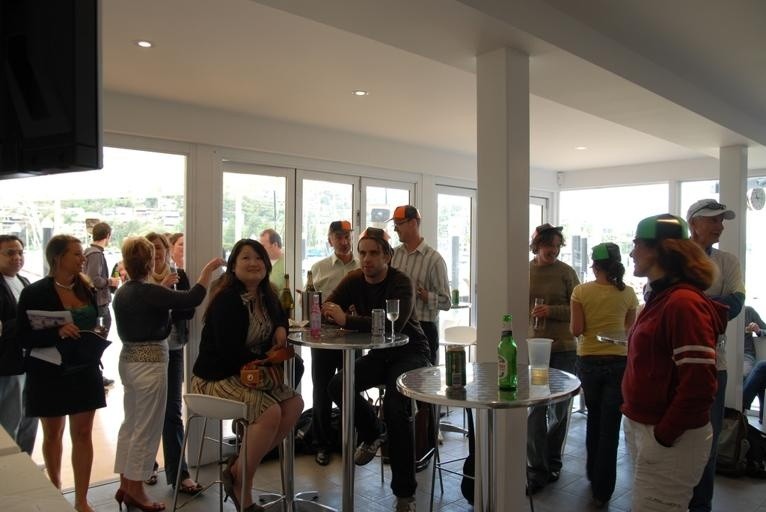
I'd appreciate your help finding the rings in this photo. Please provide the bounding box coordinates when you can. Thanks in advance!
[331,305,335,308]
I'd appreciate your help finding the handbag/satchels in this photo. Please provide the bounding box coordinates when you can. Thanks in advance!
[241,359,286,391]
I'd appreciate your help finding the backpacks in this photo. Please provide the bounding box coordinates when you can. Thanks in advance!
[715,408,751,478]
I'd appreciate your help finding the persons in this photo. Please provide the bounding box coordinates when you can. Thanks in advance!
[383,205,451,473]
[621,216,731,512]
[642,198,746,512]
[260,228,285,298]
[299,221,362,466]
[322,226,431,512]
[570,242,640,509]
[743,361,766,412]
[527,223,580,496]
[743,305,766,381]
[190,239,304,512]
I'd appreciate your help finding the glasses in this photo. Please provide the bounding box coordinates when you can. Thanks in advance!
[691,202,727,215]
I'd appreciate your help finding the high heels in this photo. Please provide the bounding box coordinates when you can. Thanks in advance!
[221,463,263,512]
[115,489,165,512]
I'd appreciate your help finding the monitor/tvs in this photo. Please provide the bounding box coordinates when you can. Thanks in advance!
[0,0,103,177]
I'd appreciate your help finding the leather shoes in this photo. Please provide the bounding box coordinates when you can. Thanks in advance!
[315,449,330,466]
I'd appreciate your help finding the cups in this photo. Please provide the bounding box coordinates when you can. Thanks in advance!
[525,336,552,369]
[301,292,323,327]
[528,366,552,397]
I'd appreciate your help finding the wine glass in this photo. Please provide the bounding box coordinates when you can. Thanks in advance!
[384,299,401,340]
[169,260,179,291]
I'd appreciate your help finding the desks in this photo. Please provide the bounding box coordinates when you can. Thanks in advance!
[398,363,586,512]
[287,327,412,509]
[449,302,473,363]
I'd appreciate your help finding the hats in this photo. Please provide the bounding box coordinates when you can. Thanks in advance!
[686,199,735,220]
[329,221,354,233]
[592,242,621,262]
[384,205,422,224]
[358,227,395,257]
[530,223,563,244]
[635,213,690,241]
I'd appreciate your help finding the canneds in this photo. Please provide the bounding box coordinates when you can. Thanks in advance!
[446,344,467,390]
[452,289,459,304]
[371,309,385,337]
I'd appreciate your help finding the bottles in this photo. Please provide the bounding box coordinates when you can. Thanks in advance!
[305,270,316,291]
[108,263,119,294]
[309,296,321,338]
[280,272,294,319]
[498,313,518,392]
[530,297,546,331]
[498,390,518,403]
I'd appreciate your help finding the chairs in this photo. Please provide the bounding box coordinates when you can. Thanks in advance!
[753,328,766,363]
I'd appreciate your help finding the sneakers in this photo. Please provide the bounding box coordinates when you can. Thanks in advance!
[392,491,416,512]
[354,421,389,466]
[172,480,203,493]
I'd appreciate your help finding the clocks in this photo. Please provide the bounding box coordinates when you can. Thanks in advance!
[748,187,765,211]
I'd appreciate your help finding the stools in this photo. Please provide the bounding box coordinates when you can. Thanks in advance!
[172,390,258,512]
[377,373,447,512]
[435,325,479,436]
